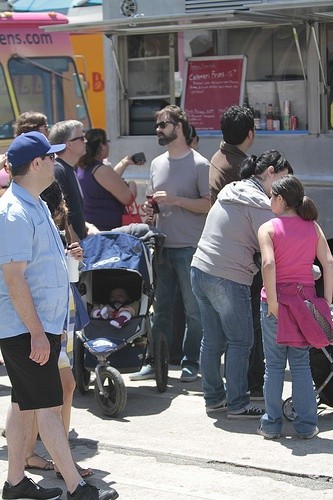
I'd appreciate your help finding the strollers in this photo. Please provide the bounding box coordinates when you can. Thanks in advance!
[281,276,333,423]
[69,223,170,418]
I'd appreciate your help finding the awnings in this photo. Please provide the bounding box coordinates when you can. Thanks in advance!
[39,11,307,98]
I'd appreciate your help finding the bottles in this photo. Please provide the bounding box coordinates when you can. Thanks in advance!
[266,104,273,130]
[272,106,282,132]
[144,182,159,215]
[254,103,262,129]
[260,102,267,130]
[65,247,80,282]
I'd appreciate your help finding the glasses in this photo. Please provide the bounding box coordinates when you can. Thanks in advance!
[69,136,85,141]
[43,153,55,159]
[105,139,110,143]
[154,120,177,128]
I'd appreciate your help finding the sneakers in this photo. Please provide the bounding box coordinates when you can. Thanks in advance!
[206,402,227,413]
[2,477,63,500]
[228,406,266,418]
[66,484,119,500]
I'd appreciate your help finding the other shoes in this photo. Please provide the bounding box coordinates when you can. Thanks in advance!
[100,306,114,320]
[248,387,264,399]
[180,365,198,380]
[300,427,319,439]
[257,425,278,438]
[130,360,168,380]
[110,315,129,329]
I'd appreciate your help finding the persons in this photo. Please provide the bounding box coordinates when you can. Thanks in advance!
[187,125,199,151]
[209,106,265,400]
[75,128,136,231]
[90,287,136,329]
[0,120,119,500]
[13,112,50,138]
[190,149,294,419]
[257,173,333,439]
[130,106,210,382]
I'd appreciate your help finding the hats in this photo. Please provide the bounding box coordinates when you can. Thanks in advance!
[8,131,66,166]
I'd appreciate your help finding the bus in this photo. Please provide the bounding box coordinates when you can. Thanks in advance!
[0,9,106,198]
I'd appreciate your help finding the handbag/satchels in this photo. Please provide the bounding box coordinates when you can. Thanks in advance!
[63,249,81,283]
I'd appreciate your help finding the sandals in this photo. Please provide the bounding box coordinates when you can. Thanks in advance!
[56,462,93,478]
[24,454,55,470]
